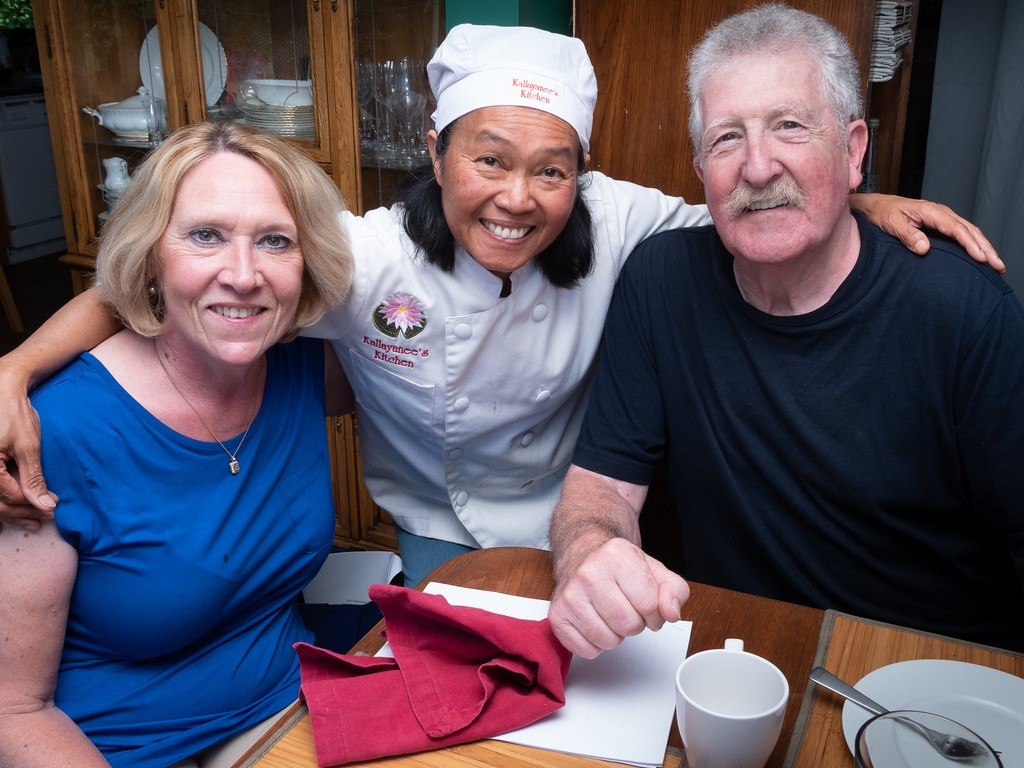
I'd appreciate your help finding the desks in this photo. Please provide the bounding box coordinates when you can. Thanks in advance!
[228,545,824,768]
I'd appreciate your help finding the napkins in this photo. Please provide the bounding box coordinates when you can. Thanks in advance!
[289,581,573,767]
[373,569,692,767]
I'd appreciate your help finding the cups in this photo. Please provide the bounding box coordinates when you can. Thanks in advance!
[854,709,1004,768]
[675,638,789,768]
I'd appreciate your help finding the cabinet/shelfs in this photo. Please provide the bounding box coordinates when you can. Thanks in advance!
[30,0,919,558]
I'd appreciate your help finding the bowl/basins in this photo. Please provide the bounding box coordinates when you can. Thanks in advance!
[246,78,313,106]
[82,86,169,138]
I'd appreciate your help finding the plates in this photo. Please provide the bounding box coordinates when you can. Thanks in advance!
[98,192,131,238]
[243,103,315,140]
[842,659,1024,768]
[138,20,228,108]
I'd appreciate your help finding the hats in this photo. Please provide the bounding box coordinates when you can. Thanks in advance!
[427,24,598,156]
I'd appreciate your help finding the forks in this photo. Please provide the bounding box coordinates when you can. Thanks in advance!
[807,666,1002,759]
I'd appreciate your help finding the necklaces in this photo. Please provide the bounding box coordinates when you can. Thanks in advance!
[153,339,261,474]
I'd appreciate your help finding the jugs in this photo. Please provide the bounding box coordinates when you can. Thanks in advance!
[102,157,131,191]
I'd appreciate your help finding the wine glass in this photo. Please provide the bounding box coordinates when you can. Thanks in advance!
[356,58,434,169]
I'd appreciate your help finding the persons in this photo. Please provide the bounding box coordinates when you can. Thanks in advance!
[0,21,1007,589]
[548,4,1024,661]
[0,123,378,768]
